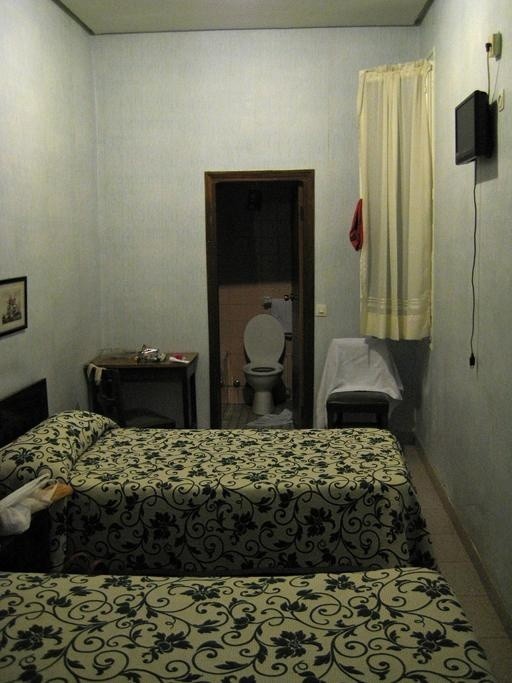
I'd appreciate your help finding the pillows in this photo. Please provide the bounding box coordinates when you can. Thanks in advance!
[0,409,120,496]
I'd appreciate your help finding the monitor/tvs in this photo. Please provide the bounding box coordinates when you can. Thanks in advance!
[455,89,490,167]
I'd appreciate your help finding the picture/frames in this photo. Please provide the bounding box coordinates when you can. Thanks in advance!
[0,277,28,337]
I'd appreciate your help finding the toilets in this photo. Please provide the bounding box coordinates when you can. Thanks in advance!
[244,312,285,417]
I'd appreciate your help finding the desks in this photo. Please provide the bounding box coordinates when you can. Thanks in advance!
[86,352,199,428]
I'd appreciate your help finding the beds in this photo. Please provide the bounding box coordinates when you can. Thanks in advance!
[0,377,441,577]
[0,567,499,682]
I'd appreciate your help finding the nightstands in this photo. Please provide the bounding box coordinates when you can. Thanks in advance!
[0,496,54,573]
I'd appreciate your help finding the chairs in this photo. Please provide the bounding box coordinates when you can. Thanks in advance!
[85,364,176,428]
[327,391,389,429]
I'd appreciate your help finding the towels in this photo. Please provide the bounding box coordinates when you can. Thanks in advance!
[245,408,294,428]
[270,299,293,339]
